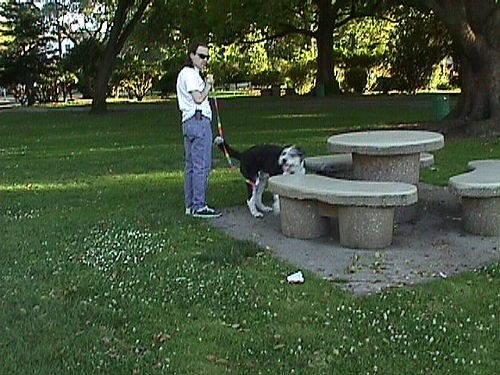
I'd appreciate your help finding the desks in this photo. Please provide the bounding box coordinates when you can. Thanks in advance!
[327,131,445,223]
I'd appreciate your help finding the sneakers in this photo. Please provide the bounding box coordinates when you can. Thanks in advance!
[193,203,223,220]
[185,205,195,216]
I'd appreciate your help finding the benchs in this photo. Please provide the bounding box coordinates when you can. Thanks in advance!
[269,174,417,250]
[304,153,434,179]
[448,159,500,235]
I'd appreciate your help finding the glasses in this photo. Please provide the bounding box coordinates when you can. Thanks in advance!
[195,51,210,61]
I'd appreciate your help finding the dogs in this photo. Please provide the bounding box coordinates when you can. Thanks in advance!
[214,136,307,219]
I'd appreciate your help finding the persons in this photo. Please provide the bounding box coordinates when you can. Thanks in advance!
[174,39,224,219]
